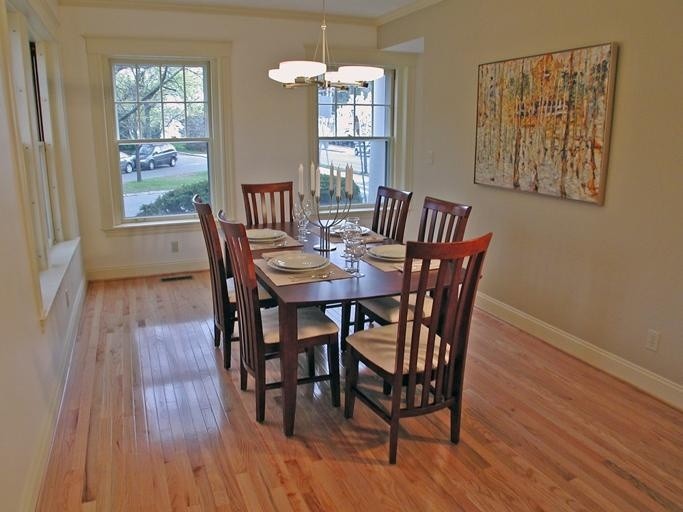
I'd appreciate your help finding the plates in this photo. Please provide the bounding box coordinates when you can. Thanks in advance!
[242,229,287,243]
[267,254,330,274]
[366,246,421,261]
[326,226,370,237]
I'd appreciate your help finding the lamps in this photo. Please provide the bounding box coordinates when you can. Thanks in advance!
[267,0,385,98]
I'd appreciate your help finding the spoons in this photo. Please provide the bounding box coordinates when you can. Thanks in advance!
[289,270,334,280]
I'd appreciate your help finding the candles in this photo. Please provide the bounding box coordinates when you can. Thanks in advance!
[295,162,353,197]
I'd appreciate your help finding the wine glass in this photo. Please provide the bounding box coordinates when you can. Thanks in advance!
[341,217,361,258]
[350,238,365,278]
[292,202,311,241]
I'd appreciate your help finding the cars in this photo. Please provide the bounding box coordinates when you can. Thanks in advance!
[119,150,135,174]
[354,138,371,158]
[131,142,177,169]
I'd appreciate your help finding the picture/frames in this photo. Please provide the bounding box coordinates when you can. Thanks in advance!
[470,41,619,207]
